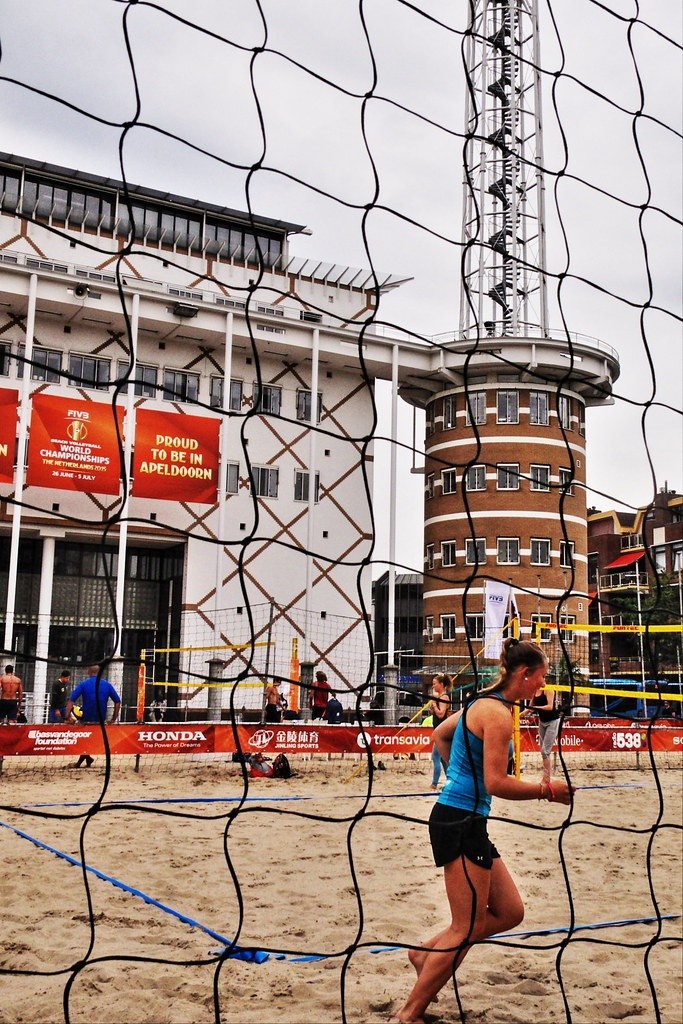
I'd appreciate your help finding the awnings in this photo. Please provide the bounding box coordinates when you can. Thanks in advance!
[603,551,646,570]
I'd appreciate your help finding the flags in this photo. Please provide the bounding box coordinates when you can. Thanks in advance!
[484,581,510,659]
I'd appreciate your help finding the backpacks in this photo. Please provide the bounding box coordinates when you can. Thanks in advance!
[273,754,290,779]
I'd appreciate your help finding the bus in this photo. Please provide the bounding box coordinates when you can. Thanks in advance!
[589,678,674,718]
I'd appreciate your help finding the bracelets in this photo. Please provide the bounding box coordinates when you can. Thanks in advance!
[538,782,554,802]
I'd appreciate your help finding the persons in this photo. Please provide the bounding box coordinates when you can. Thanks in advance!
[662,700,680,718]
[308,671,336,720]
[66,666,120,724]
[263,678,287,723]
[0,665,22,723]
[518,686,560,784]
[49,670,83,724]
[395,635,576,1024]
[429,673,451,789]
[149,690,167,722]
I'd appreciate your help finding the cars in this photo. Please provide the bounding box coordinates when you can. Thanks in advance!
[375,690,423,724]
